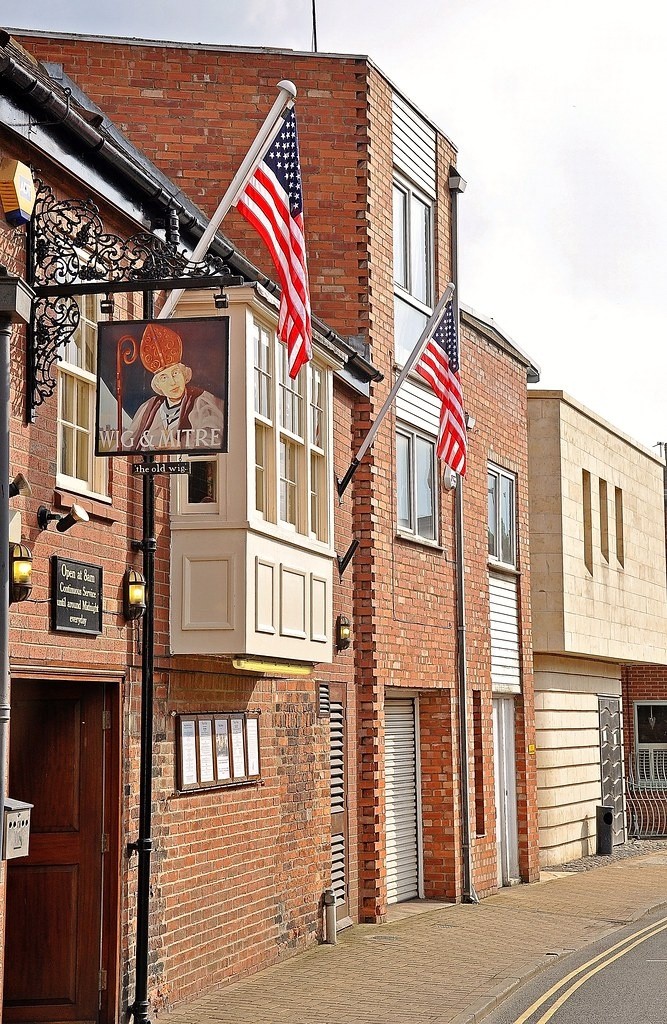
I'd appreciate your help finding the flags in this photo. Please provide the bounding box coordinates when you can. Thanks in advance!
[416,298,467,483]
[234,105,312,379]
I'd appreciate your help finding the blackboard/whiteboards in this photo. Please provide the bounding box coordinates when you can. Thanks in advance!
[49,555,104,637]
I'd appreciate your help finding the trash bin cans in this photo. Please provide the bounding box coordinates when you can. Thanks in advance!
[596,805,614,856]
